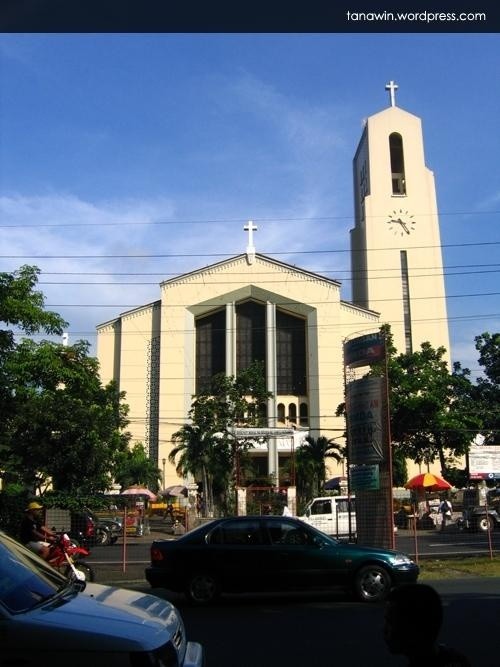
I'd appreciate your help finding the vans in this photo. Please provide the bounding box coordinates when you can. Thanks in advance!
[296,494,358,544]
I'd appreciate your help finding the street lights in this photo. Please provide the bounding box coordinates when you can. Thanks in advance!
[162,459,166,490]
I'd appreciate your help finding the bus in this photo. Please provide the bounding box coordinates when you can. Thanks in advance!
[93,494,150,536]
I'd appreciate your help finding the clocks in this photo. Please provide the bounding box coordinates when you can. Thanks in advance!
[386,208,417,238]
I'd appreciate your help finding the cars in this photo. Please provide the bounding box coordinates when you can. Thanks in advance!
[468,505,500,534]
[75,506,123,547]
[145,516,420,608]
[0,529,208,667]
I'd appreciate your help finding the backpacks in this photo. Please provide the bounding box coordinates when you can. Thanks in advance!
[441,501,449,512]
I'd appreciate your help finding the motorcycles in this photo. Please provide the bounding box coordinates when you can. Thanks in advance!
[47,534,95,583]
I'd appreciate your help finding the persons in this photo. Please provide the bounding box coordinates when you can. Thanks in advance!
[478,479,497,505]
[162,496,174,523]
[437,495,453,533]
[273,490,288,516]
[196,494,205,518]
[221,497,231,518]
[19,502,59,560]
[407,491,419,531]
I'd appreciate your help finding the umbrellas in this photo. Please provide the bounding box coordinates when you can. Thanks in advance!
[321,477,351,496]
[120,483,158,504]
[402,471,453,530]
[161,484,188,514]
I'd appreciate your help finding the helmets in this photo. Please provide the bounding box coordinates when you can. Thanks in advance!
[24,502,44,512]
